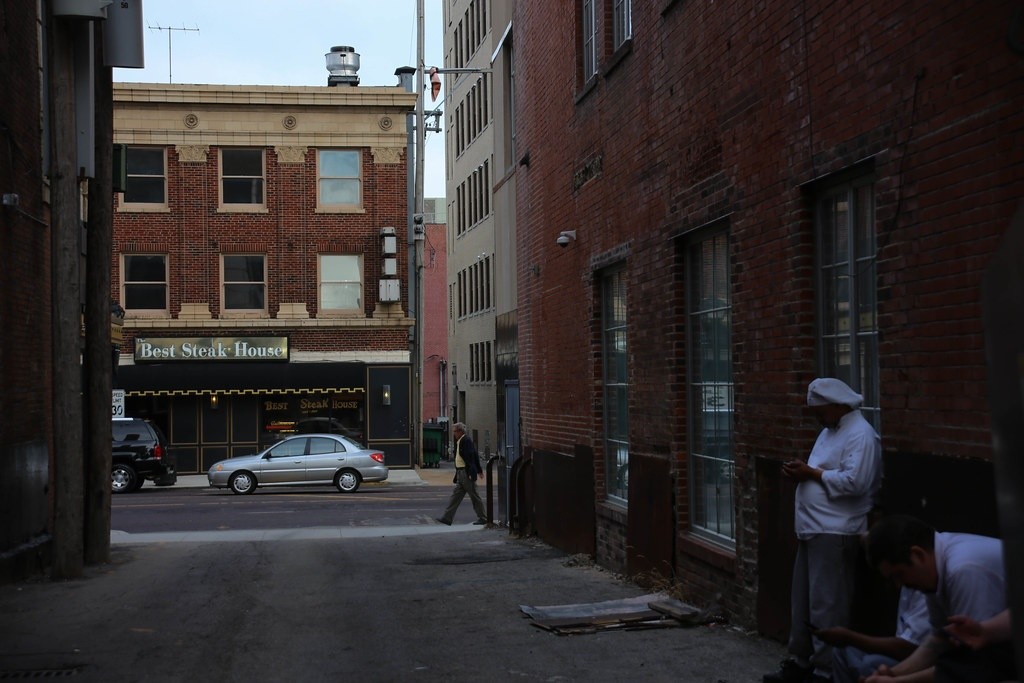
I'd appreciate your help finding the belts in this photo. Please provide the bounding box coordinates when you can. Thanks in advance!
[456,467,465,470]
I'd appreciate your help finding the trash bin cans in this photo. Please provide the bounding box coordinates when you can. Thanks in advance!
[423,422,445,469]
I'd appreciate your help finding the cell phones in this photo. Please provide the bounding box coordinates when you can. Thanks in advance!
[804,620,820,631]
[936,629,974,654]
[783,457,795,462]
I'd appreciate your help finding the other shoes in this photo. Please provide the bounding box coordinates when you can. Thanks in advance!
[763,658,834,683]
[473,518,487,525]
[436,518,452,526]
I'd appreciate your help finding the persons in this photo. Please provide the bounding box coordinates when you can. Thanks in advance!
[816,515,1024,683]
[760,378,880,683]
[436,422,488,525]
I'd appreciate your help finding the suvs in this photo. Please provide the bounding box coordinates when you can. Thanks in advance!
[702,381,734,485]
[110,417,165,495]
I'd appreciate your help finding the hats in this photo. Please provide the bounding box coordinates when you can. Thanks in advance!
[806,377,864,412]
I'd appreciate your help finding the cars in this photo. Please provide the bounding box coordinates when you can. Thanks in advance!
[296,416,349,437]
[207,434,389,495]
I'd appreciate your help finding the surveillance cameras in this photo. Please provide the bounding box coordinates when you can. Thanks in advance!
[558,235,569,247]
